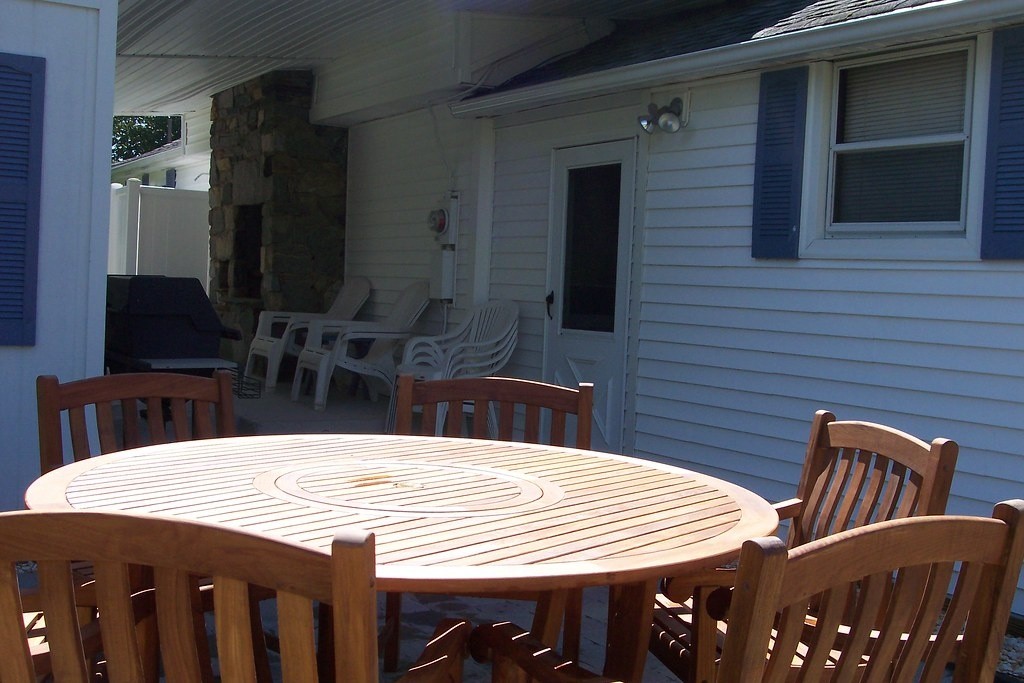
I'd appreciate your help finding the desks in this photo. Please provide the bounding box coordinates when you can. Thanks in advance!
[24,433,778,683]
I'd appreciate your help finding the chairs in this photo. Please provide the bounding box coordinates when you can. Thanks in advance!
[0,277,1024,683]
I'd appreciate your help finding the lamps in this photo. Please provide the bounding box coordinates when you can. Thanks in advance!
[638,91,691,135]
